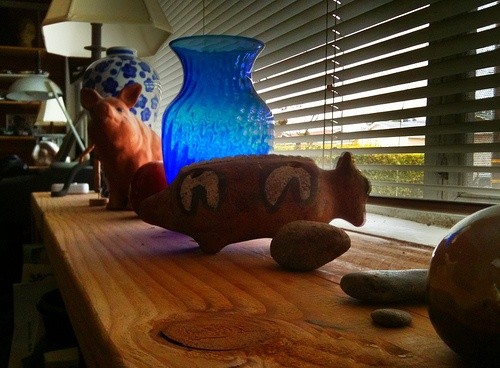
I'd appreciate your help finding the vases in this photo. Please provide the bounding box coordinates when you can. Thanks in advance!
[162,36,274,189]
[83,45,161,130]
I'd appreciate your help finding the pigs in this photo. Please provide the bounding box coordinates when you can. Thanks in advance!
[78,82,163,211]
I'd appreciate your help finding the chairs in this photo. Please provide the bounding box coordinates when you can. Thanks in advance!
[48,163,95,188]
[0,160,27,290]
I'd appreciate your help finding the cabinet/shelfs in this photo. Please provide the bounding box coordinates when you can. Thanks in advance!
[0,46,71,170]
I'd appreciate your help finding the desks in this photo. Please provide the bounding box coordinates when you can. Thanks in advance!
[30,190,500,368]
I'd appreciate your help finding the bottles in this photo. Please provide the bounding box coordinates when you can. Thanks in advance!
[161,35,274,186]
[82,47,161,126]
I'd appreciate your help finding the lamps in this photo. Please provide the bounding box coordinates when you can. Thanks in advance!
[6,77,91,162]
[41,0,171,196]
[34,98,68,136]
